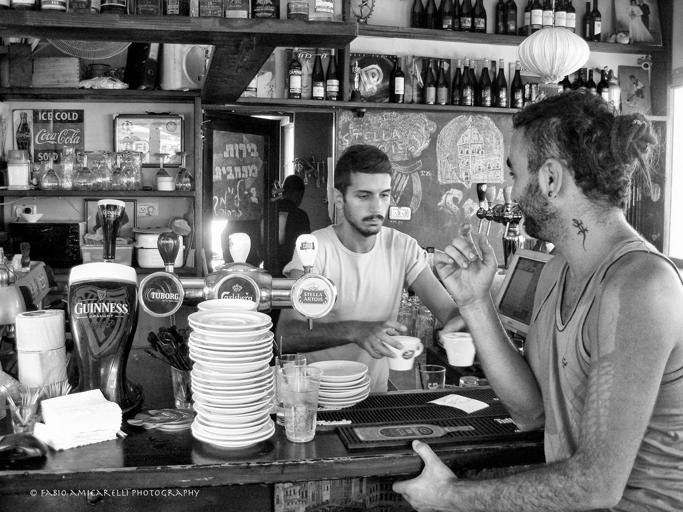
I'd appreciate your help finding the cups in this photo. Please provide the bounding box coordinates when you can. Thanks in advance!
[275,352,308,427]
[277,365,323,445]
[386,334,423,371]
[458,374,482,389]
[441,332,477,367]
[413,364,447,392]
[8,402,36,437]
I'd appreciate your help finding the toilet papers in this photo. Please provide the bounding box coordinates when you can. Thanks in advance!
[19,347,68,386]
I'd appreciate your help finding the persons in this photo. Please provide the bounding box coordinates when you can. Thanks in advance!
[259,176,311,278]
[392,90,682,511]
[275,145,467,393]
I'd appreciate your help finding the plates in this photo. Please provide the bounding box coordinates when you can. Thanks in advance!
[306,359,373,403]
[185,310,277,450]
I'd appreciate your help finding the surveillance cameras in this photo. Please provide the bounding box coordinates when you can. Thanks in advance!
[637,54,652,71]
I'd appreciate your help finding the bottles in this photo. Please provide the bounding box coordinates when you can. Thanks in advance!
[425,0,438,29]
[597,70,610,102]
[453,0,462,31]
[411,0,426,29]
[572,69,586,94]
[490,60,498,107]
[531,0,543,33]
[586,69,597,96]
[524,0,533,36]
[473,0,487,33]
[203,233,272,316]
[554,0,566,28]
[390,57,404,103]
[424,59,437,104]
[156,177,176,191]
[582,2,594,40]
[411,56,422,102]
[608,69,620,85]
[438,0,454,30]
[566,0,576,32]
[403,57,413,103]
[461,0,474,32]
[437,60,450,105]
[461,60,474,106]
[452,60,463,105]
[496,0,507,34]
[311,48,325,100]
[328,48,339,100]
[289,49,302,99]
[542,0,554,29]
[7,150,31,190]
[505,0,517,35]
[469,60,480,106]
[16,112,32,159]
[497,60,508,107]
[561,76,572,96]
[591,0,602,42]
[479,60,494,106]
[511,65,525,108]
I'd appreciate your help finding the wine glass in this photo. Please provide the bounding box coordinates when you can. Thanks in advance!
[37,144,141,194]
[153,151,171,191]
[176,151,193,192]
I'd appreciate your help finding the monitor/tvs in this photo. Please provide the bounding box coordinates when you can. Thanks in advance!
[493,248,556,339]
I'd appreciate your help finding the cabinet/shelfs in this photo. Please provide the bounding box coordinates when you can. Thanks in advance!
[1,1,359,100]
[347,0,668,120]
[0,97,201,283]
